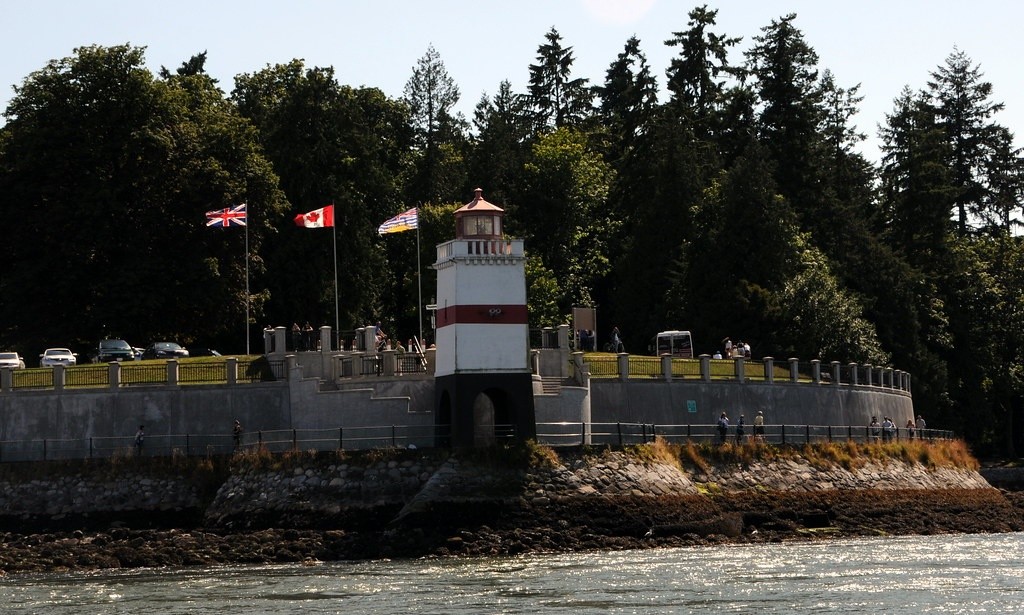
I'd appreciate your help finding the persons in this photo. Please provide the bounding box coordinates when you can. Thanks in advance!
[755,411,764,434]
[738,415,744,445]
[263,325,275,339]
[882,416,897,442]
[724,340,752,363]
[233,420,240,445]
[135,425,145,456]
[375,321,387,346]
[915,415,926,437]
[292,321,313,351]
[906,420,914,436]
[713,351,722,359]
[870,417,880,443]
[719,412,729,442]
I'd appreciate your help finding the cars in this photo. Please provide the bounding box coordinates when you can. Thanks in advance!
[92,335,135,363]
[132,342,191,360]
[38,348,79,367]
[0,353,26,370]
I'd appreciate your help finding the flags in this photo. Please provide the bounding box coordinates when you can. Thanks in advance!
[378,208,418,234]
[294,205,334,228]
[205,204,246,227]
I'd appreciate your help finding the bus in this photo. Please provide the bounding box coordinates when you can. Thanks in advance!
[648,330,693,359]
